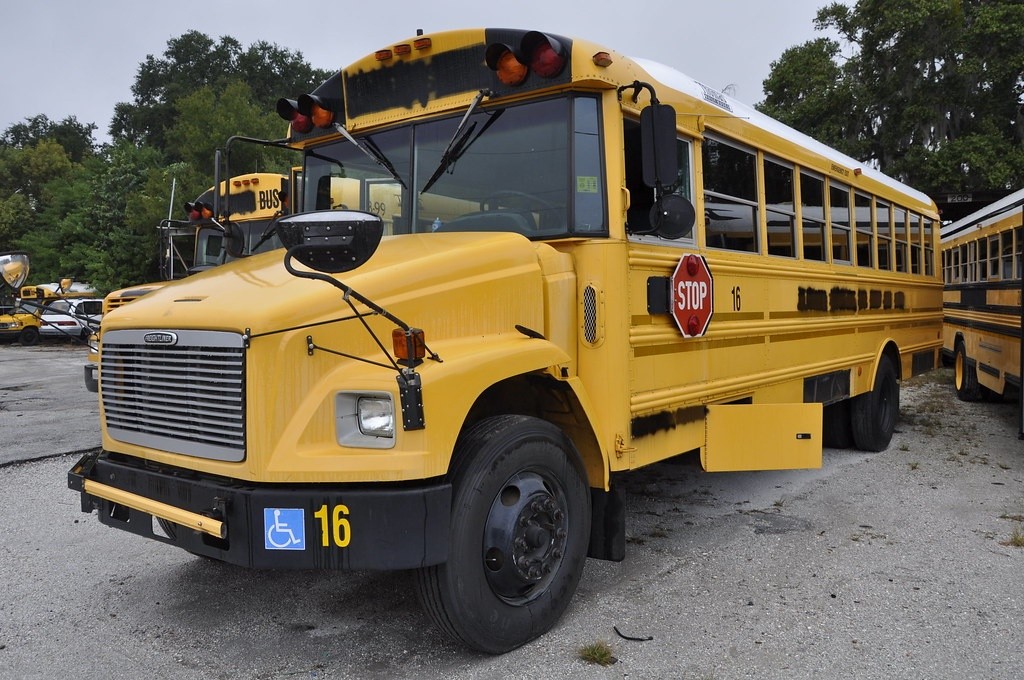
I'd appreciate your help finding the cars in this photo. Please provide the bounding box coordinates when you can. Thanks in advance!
[38,298,104,343]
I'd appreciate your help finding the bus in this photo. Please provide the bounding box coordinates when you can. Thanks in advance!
[84,167,543,395]
[66,24,946,661]
[696,199,933,276]
[0,283,96,346]
[940,187,1024,404]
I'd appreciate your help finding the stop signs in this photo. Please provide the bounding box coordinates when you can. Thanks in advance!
[671,252,715,340]
